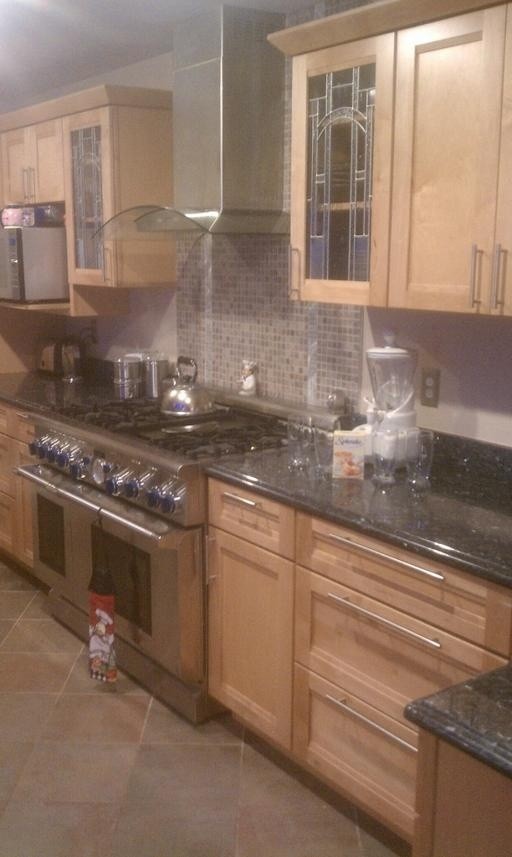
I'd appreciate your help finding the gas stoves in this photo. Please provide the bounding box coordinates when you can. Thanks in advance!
[59,393,299,468]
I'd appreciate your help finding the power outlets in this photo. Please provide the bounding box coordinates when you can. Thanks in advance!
[91,319,96,343]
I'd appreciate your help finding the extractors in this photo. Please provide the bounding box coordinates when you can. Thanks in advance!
[90,3,290,243]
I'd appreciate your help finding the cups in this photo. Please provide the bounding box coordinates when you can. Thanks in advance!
[402,430,434,490]
[285,413,312,472]
[315,414,343,476]
[371,426,398,483]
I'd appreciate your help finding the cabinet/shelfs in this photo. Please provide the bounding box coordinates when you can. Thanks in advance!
[205,476,512,850]
[266,0,512,316]
[0,83,177,318]
[0,401,35,576]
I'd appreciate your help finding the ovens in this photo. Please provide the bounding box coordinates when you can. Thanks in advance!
[14,420,207,682]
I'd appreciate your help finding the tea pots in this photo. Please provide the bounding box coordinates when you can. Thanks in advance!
[161,356,217,416]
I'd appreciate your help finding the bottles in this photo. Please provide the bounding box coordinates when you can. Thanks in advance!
[1,204,25,226]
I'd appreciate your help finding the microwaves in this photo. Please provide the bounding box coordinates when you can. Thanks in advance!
[0,225,72,305]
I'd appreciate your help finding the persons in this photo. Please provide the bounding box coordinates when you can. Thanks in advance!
[235,359,259,396]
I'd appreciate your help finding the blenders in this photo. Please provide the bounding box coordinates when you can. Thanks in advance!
[366,328,417,461]
